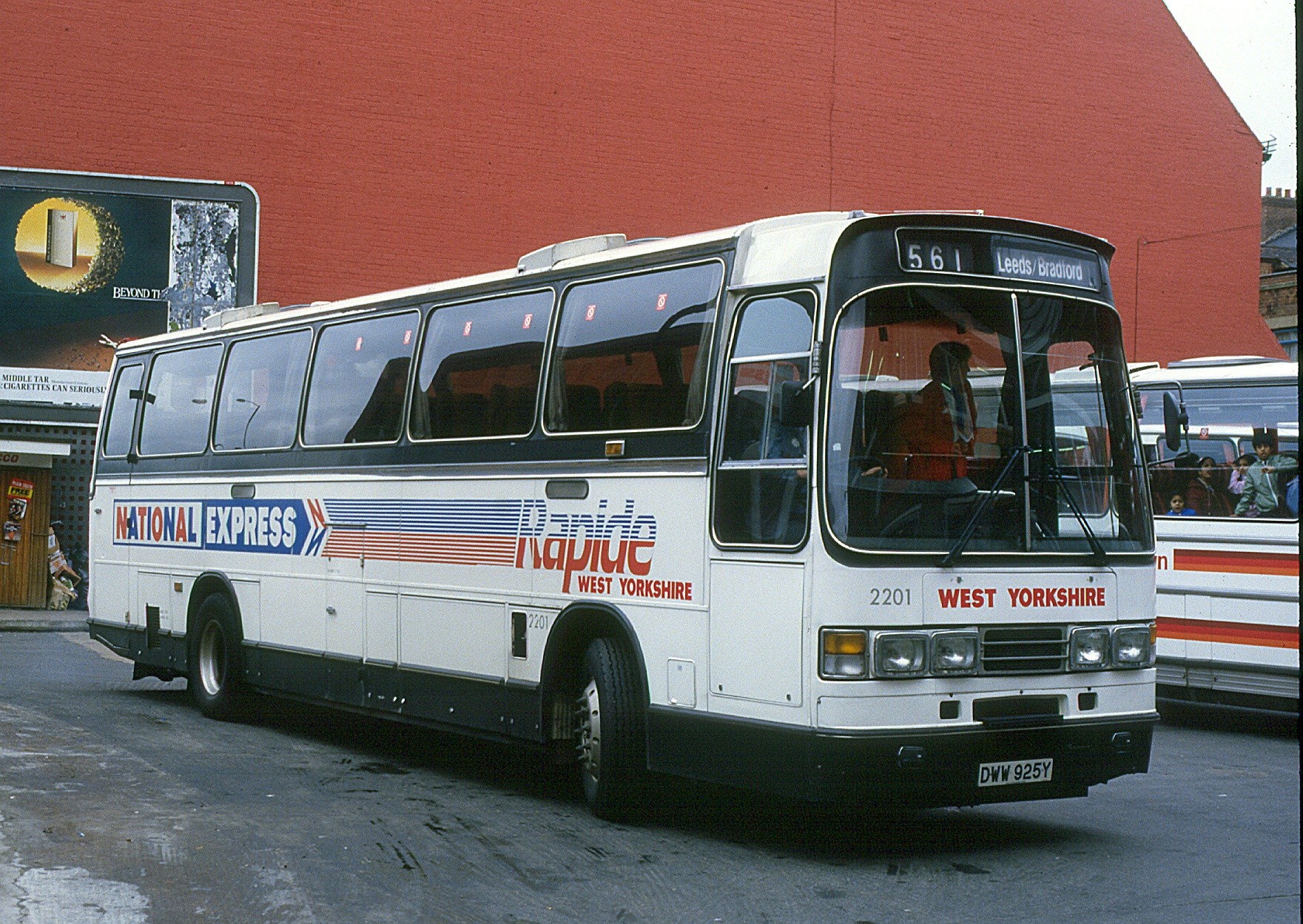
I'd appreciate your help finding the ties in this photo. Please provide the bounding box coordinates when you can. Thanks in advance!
[954,391,964,434]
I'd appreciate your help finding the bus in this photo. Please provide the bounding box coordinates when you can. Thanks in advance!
[84,204,1193,820]
[733,352,1300,732]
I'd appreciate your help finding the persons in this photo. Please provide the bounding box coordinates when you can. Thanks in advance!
[1162,432,1300,518]
[890,340,979,502]
